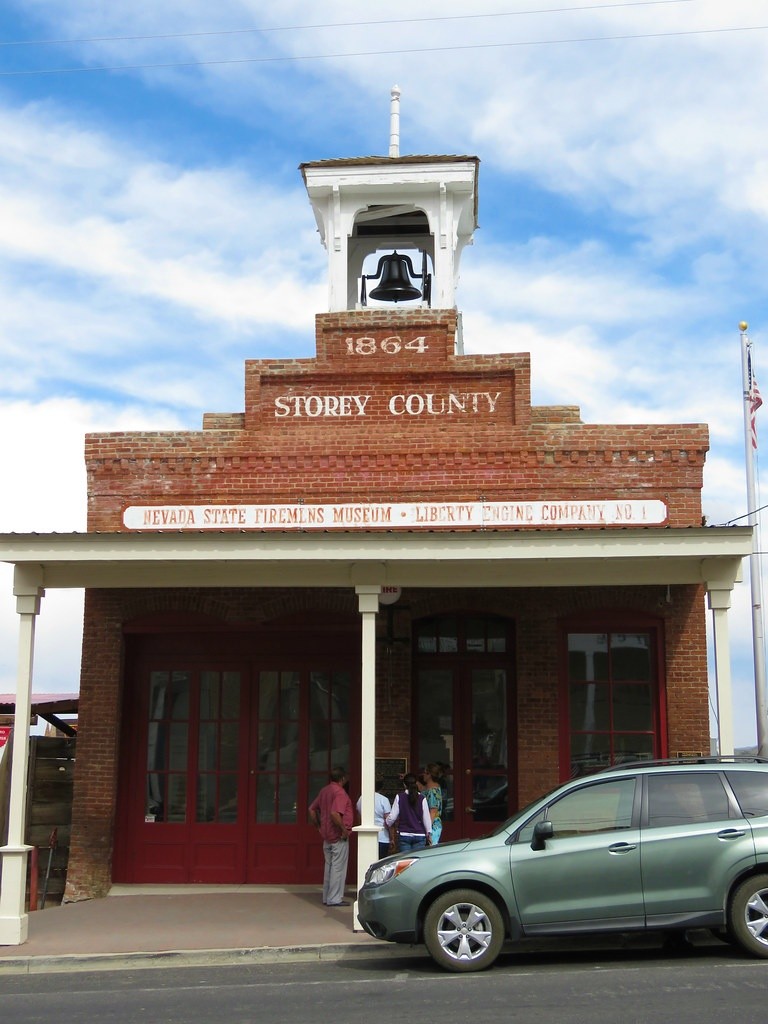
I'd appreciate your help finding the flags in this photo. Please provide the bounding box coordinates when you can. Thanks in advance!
[742,343,763,449]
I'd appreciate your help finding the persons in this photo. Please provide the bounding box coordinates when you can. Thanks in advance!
[308,766,353,906]
[357,763,442,860]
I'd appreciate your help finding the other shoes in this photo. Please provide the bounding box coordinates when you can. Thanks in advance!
[324,900,351,906]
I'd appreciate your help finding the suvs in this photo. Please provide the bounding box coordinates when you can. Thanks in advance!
[356,752,768,971]
[443,753,656,822]
